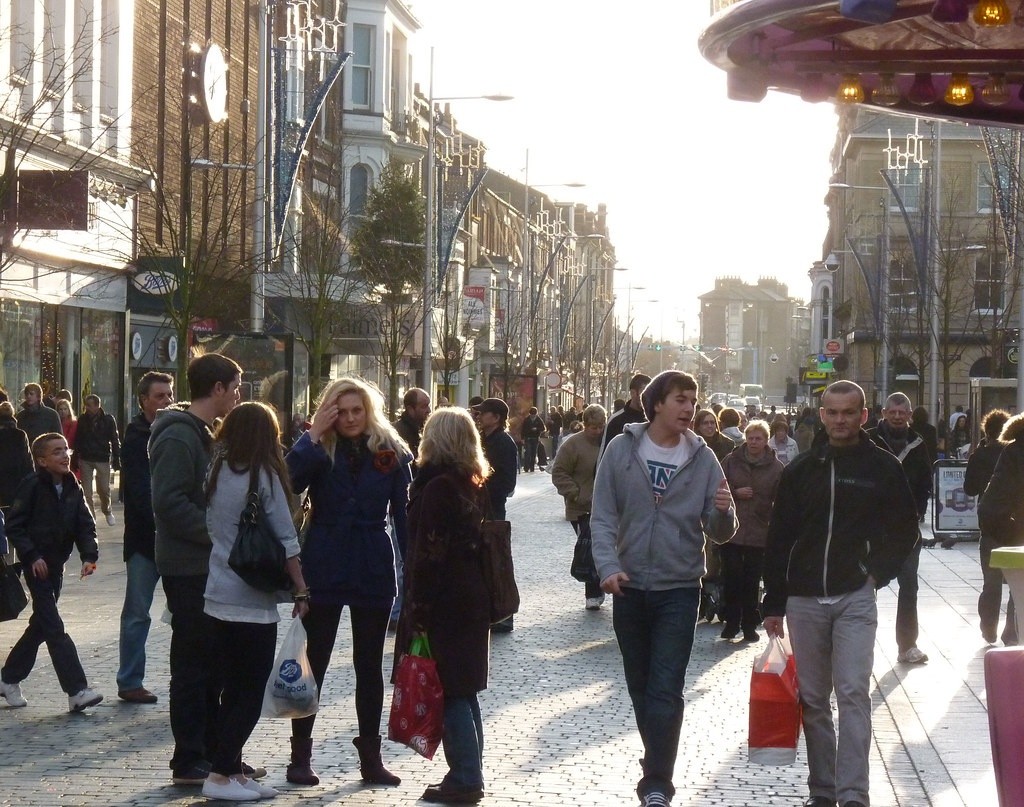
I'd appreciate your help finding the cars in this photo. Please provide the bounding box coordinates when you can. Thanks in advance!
[707,393,763,415]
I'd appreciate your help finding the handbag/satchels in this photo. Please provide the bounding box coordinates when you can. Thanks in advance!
[387,630,442,760]
[291,495,311,534]
[228,461,294,592]
[481,480,520,627]
[746,628,803,768]
[260,612,320,720]
[570,523,597,584]
[0,556,29,623]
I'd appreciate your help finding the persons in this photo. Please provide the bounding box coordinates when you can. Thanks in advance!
[0,354,1024,807]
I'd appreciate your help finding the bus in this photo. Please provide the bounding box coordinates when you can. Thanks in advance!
[738,383,765,403]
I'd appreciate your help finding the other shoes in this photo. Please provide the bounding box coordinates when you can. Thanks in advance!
[743,627,760,643]
[106,512,117,527]
[643,791,671,807]
[118,686,159,702]
[425,779,485,802]
[721,621,741,639]
[806,795,837,807]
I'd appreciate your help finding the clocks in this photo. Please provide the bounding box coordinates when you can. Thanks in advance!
[200,44,230,124]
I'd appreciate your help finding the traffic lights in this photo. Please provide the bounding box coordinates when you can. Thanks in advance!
[692,344,704,352]
[655,344,662,352]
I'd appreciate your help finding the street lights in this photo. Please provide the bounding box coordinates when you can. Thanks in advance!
[595,279,660,422]
[534,205,606,413]
[189,158,264,337]
[677,320,686,371]
[489,144,587,376]
[565,255,629,409]
[378,44,516,397]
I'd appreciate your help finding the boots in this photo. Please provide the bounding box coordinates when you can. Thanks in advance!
[286,736,319,783]
[351,734,401,785]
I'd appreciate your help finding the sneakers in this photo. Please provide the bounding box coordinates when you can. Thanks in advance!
[898,648,928,663]
[0,677,29,709]
[240,763,266,777]
[201,774,261,800]
[173,758,229,785]
[586,596,604,611]
[242,777,281,799]
[67,688,104,713]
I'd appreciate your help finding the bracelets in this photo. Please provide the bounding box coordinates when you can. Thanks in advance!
[290,591,310,602]
